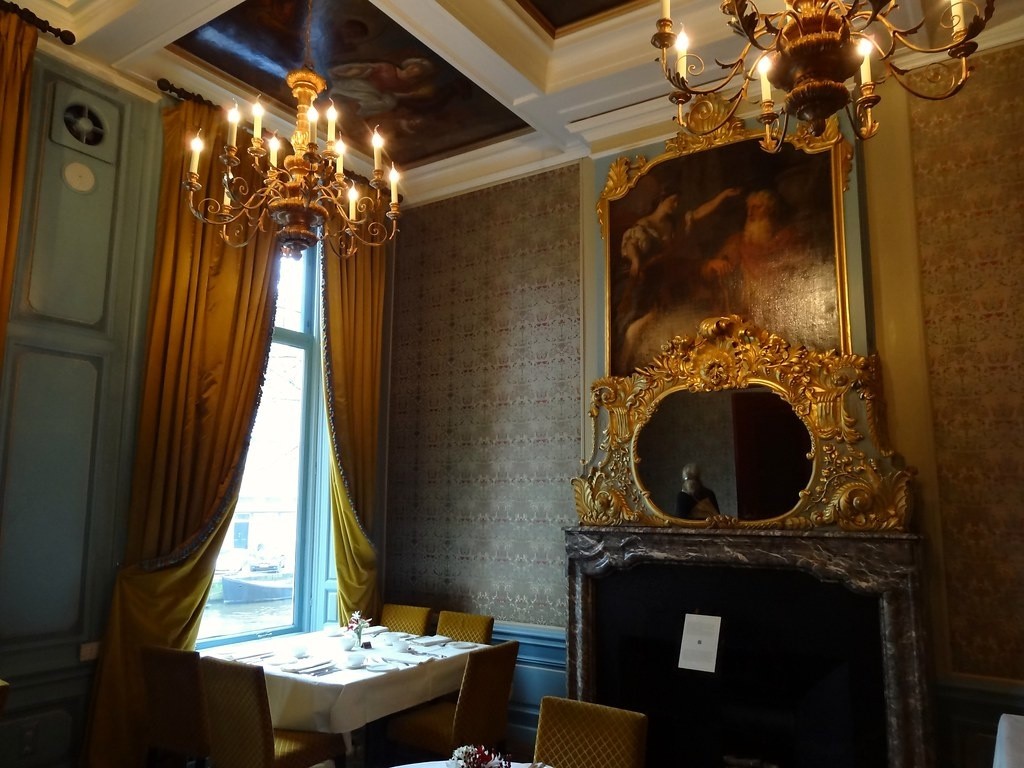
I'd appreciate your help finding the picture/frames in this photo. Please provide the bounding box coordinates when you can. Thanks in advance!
[596,90,855,378]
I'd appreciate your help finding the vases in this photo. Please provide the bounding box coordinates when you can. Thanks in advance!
[352,629,363,647]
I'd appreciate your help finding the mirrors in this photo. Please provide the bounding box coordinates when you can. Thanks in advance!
[570,315,918,531]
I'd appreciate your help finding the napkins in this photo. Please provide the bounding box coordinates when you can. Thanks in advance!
[361,626,389,638]
[412,634,453,647]
[280,656,341,677]
[226,651,276,663]
[381,652,436,665]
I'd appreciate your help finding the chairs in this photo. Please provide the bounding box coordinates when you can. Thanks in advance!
[386,640,520,761]
[436,610,496,645]
[534,696,646,768]
[137,644,348,768]
[379,603,432,636]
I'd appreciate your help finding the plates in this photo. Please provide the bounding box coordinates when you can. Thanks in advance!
[366,664,398,671]
[449,642,476,648]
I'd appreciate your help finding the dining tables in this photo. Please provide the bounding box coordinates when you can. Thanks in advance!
[194,631,495,768]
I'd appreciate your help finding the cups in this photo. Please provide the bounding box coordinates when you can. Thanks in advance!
[339,638,355,650]
[393,642,409,649]
[382,635,399,645]
[347,653,368,666]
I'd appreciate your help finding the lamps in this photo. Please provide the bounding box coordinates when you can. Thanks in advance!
[180,0,402,259]
[650,0,997,154]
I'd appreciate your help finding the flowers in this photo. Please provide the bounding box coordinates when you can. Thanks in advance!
[446,744,512,768]
[348,610,372,633]
